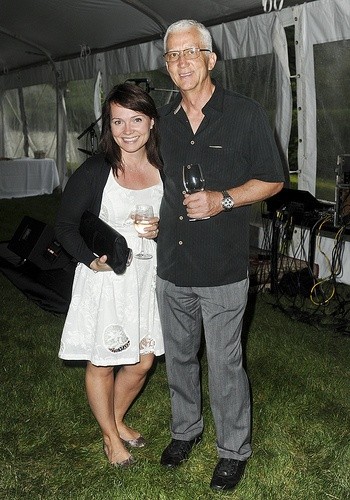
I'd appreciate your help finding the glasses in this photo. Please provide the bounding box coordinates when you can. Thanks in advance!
[161,48,211,62]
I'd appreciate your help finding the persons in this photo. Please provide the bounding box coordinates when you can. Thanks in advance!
[147,20,286,491]
[56,83,164,466]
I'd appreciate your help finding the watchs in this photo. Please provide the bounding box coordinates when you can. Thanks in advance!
[221,191,234,212]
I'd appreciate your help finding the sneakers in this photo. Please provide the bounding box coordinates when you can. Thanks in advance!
[210,458,247,491]
[160,434,203,468]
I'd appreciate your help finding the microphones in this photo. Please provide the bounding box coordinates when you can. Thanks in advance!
[127,77,152,85]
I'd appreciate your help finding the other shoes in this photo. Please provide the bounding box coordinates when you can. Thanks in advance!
[103,444,138,470]
[119,430,148,450]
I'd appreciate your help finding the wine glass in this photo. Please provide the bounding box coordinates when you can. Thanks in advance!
[133,203,154,260]
[183,162,210,221]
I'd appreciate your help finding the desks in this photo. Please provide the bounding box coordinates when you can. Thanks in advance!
[0,158,62,200]
[262,212,350,294]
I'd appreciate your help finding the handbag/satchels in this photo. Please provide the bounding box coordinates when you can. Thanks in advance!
[79,210,132,275]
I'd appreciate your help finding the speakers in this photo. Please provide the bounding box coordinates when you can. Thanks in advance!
[7,215,51,262]
